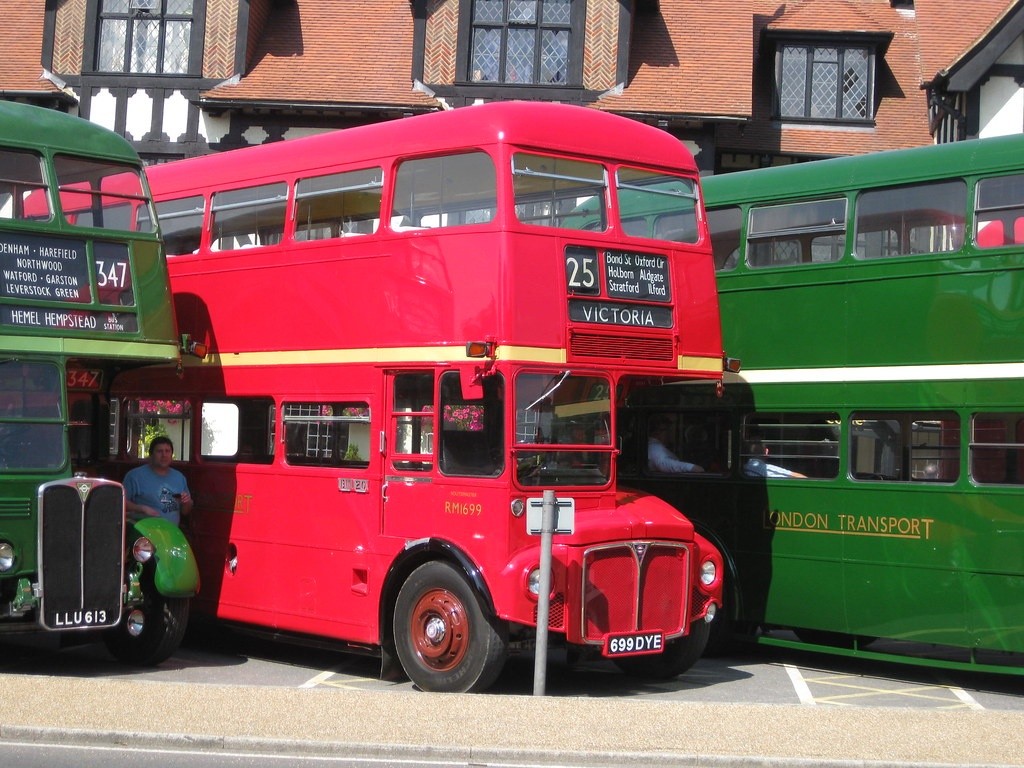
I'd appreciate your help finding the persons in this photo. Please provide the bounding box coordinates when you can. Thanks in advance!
[120,435,194,532]
[646,414,705,473]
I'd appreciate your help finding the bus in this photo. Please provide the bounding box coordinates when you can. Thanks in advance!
[0,100,200,667]
[557,132,1022,682]
[17,102,744,694]
